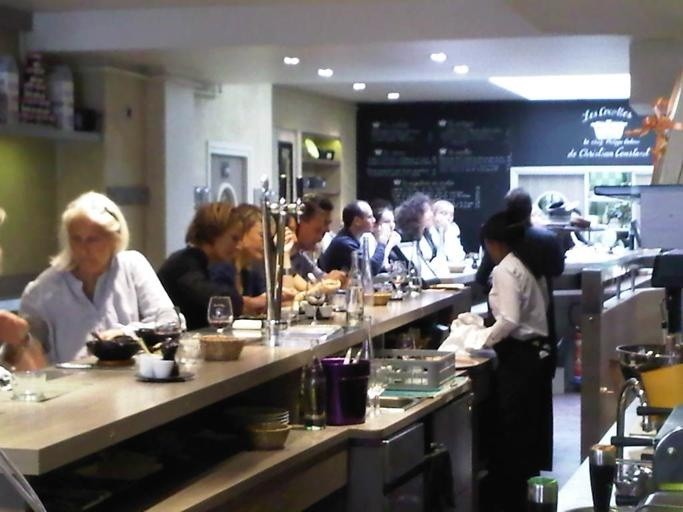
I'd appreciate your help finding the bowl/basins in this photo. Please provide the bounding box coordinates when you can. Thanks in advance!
[249,422,290,451]
[446,262,466,274]
[198,334,248,359]
[84,338,138,360]
[134,329,180,347]
[73,108,100,131]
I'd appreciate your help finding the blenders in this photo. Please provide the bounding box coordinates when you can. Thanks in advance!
[612,247,682,413]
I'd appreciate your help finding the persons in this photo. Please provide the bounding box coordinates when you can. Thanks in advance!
[471,189,564,512]
[156,192,466,332]
[1,190,187,368]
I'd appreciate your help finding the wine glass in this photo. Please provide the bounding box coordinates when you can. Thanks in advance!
[365,360,389,418]
[153,306,182,329]
[206,295,233,333]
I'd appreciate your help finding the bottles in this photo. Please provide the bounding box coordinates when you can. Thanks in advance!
[0,53,20,125]
[159,337,181,376]
[281,232,420,331]
[49,51,76,129]
[296,335,329,429]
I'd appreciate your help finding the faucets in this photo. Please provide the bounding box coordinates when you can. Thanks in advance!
[614,377,653,483]
[259,174,305,347]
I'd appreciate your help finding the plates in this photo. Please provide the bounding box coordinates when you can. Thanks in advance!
[227,399,291,426]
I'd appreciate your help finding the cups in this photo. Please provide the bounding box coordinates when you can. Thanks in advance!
[136,357,174,377]
[526,477,558,512]
[9,369,46,402]
[589,441,614,509]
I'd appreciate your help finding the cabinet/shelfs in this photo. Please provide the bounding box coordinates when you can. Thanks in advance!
[294,128,344,235]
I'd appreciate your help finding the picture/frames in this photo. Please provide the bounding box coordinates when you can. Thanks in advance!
[278,140,295,206]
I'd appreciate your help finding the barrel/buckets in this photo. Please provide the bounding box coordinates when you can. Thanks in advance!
[614,343,682,432]
